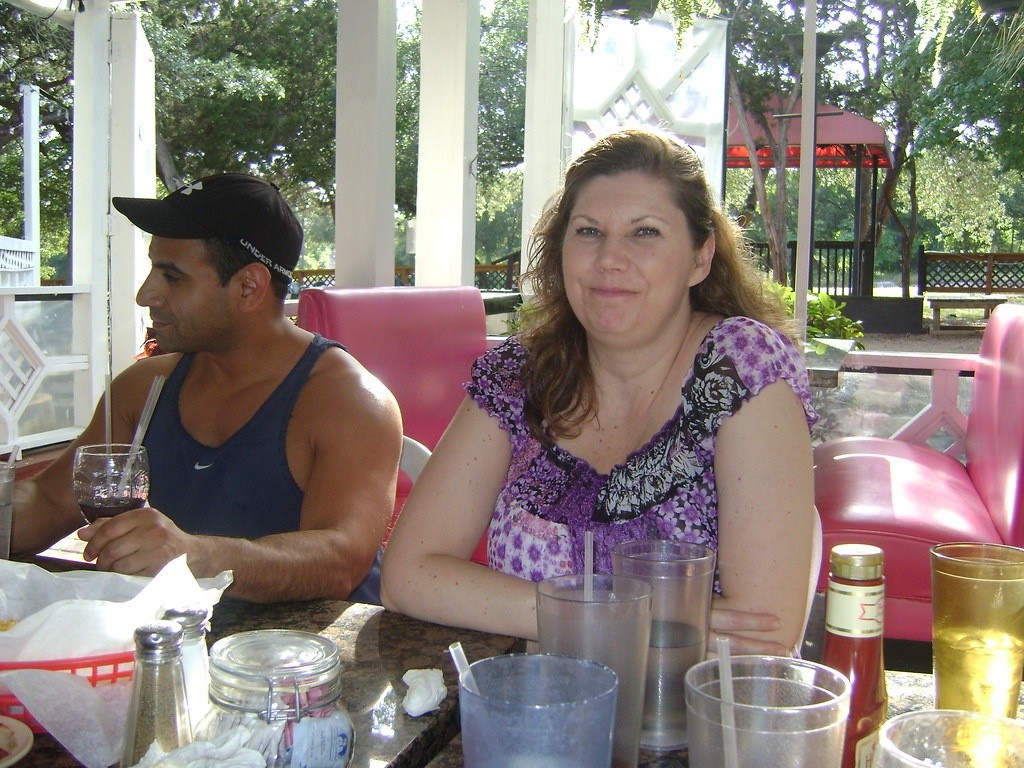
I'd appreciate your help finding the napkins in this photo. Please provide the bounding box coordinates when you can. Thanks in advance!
[132,725,267,768]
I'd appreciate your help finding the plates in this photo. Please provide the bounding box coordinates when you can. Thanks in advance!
[0,713,34,768]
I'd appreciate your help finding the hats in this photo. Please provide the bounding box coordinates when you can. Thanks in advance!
[112,174,303,282]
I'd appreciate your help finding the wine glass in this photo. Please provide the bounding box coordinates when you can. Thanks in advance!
[72,443,152,525]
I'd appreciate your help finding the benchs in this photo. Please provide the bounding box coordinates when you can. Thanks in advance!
[927,296,1007,336]
[812,302,1024,642]
[298,285,489,567]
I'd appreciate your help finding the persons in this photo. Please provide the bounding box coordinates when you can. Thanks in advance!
[6,173,404,602]
[379,130,820,664]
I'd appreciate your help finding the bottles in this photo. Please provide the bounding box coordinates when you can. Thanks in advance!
[119,619,194,768]
[193,629,357,768]
[816,543,889,768]
[154,598,211,730]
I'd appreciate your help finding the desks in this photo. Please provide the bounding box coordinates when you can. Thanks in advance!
[0,555,1024,768]
[803,338,856,388]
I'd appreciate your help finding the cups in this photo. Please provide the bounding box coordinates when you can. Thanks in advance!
[535,573,653,768]
[0,461,16,560]
[929,542,1024,719]
[457,651,620,768]
[683,654,853,768]
[609,539,717,753]
[870,708,1024,768]
